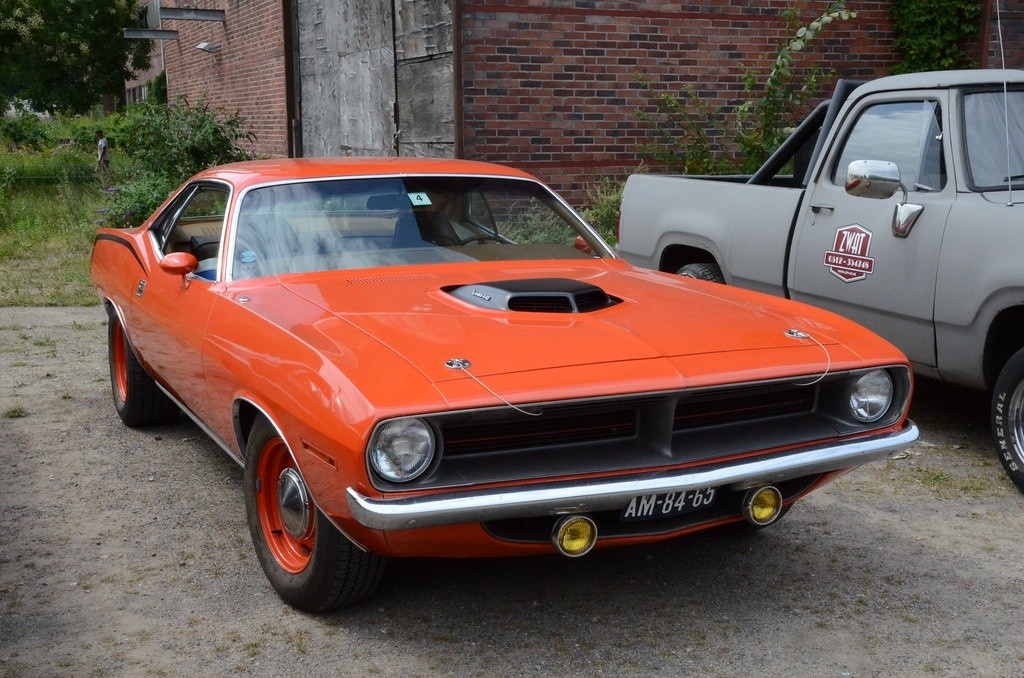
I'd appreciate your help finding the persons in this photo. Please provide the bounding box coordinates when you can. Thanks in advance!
[94,129,108,172]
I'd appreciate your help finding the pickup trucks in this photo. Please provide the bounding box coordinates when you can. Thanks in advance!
[616,69,1024,495]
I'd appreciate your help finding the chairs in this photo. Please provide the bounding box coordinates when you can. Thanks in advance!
[235,214,303,259]
[391,211,461,248]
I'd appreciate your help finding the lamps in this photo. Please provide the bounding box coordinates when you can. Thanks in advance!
[196,42,215,53]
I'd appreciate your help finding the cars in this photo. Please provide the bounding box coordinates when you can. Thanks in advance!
[91,156,919,613]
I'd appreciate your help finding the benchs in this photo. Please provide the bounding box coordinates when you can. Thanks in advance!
[165,216,397,254]
